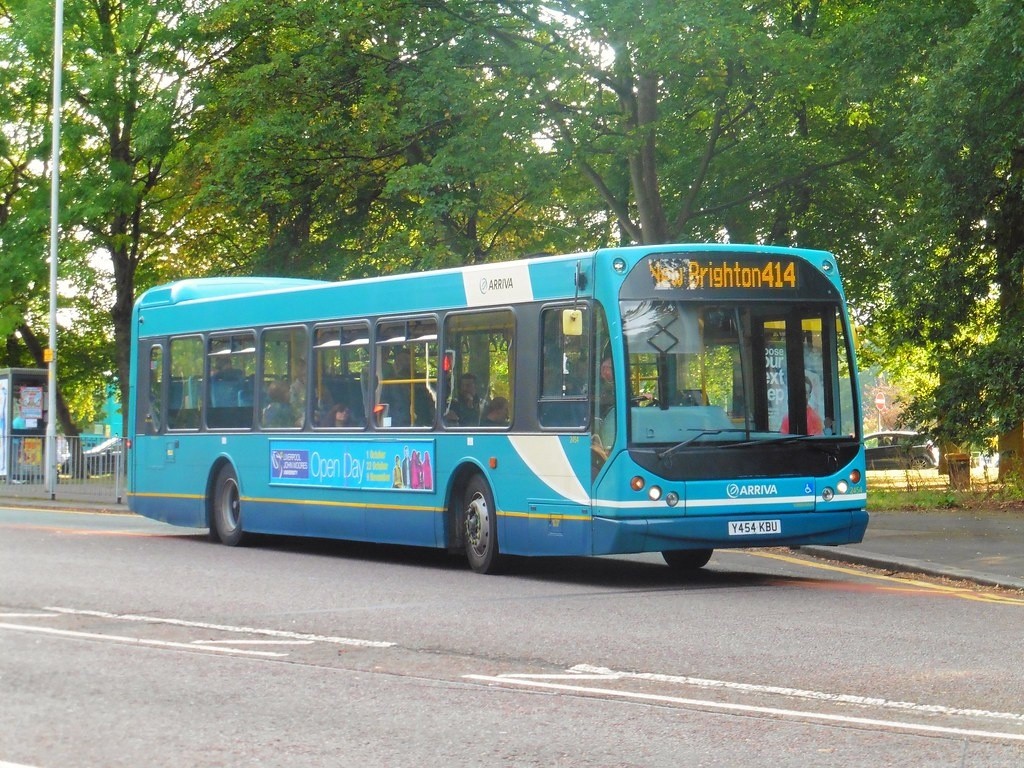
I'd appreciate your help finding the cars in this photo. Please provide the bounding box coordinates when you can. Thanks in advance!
[864,431,939,470]
[62,438,122,474]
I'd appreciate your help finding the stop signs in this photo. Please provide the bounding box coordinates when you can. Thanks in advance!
[875,393,885,410]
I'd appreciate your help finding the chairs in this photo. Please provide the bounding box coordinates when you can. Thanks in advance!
[210,371,243,428]
[325,371,366,428]
[235,373,279,427]
[884,438,891,446]
[189,374,203,428]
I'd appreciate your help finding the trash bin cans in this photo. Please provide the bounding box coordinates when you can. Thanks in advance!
[945,454,971,490]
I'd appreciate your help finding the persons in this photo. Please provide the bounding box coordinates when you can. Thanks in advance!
[210,356,235,382]
[360,346,435,428]
[323,404,353,427]
[599,358,653,418]
[444,374,508,427]
[392,446,432,489]
[262,357,307,428]
[780,376,822,436]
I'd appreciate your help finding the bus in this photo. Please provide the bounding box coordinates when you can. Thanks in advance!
[126,243,869,574]
